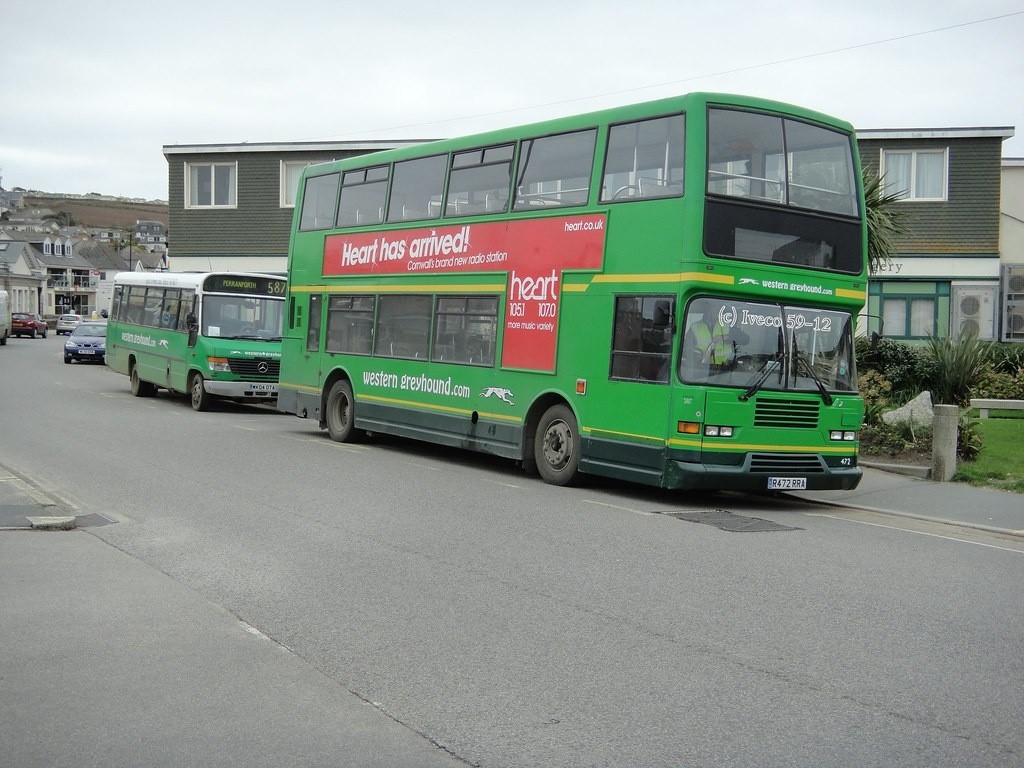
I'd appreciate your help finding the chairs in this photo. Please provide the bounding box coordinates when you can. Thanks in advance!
[248,320,263,336]
[638,177,683,197]
[307,325,496,365]
[315,193,508,227]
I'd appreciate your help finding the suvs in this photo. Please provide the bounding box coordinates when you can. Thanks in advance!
[12,312,48,339]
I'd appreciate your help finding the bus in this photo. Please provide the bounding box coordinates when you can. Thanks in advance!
[105,272,288,411]
[277,92,869,491]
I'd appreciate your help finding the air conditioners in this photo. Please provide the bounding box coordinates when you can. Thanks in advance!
[951,281,998,342]
[1001,263,1024,342]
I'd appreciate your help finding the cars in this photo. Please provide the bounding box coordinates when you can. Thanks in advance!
[56,314,82,335]
[64,322,107,364]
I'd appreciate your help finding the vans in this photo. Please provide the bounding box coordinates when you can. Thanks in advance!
[0,290,12,345]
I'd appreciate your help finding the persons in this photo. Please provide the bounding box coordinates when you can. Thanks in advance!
[685,299,751,383]
